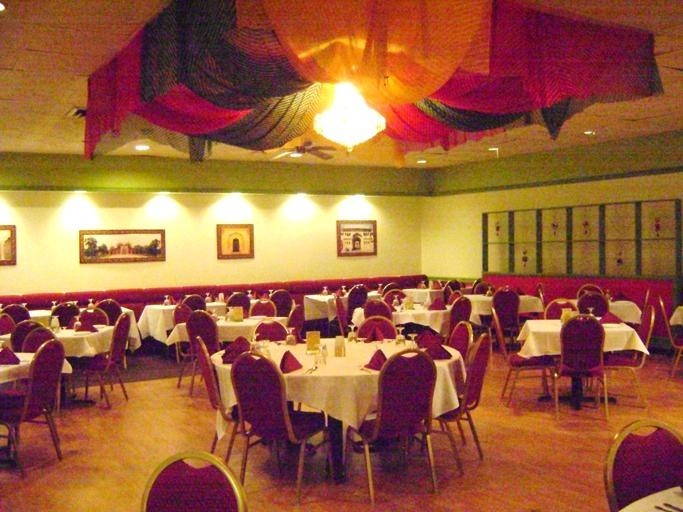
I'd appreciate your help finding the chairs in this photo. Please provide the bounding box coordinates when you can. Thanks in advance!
[0,278,683,512]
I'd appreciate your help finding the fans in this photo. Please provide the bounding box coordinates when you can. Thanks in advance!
[268,145,337,160]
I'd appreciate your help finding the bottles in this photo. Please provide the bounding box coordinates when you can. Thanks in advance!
[50,316,60,334]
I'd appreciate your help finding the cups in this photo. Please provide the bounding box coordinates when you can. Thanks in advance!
[403,295,414,312]
[559,306,573,323]
[224,306,244,321]
[334,335,345,357]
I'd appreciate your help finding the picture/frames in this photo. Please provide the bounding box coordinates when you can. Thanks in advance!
[336,220,377,256]
[0,225,16,265]
[216,224,254,259]
[78,230,166,264]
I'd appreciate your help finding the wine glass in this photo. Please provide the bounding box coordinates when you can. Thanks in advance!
[162,295,170,304]
[341,284,346,295]
[485,286,492,298]
[86,298,93,308]
[21,302,26,307]
[248,327,419,375]
[204,288,273,304]
[322,286,329,297]
[391,294,399,308]
[586,307,596,320]
[72,315,81,332]
[375,281,383,295]
[0,303,3,312]
[50,301,58,310]
